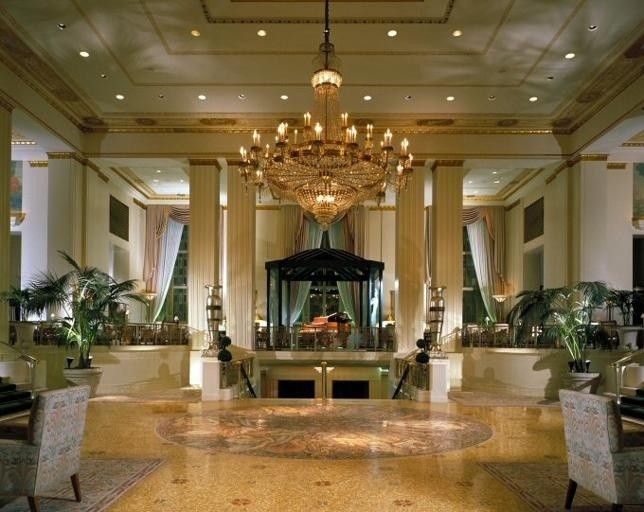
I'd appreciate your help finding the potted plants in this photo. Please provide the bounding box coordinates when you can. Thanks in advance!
[608,285,644,349]
[508,278,616,393]
[31,249,150,399]
[0,272,57,347]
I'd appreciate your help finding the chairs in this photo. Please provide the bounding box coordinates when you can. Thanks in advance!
[558,388,644,512]
[0,385,91,512]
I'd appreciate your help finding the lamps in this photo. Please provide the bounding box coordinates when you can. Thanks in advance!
[237,0,413,234]
